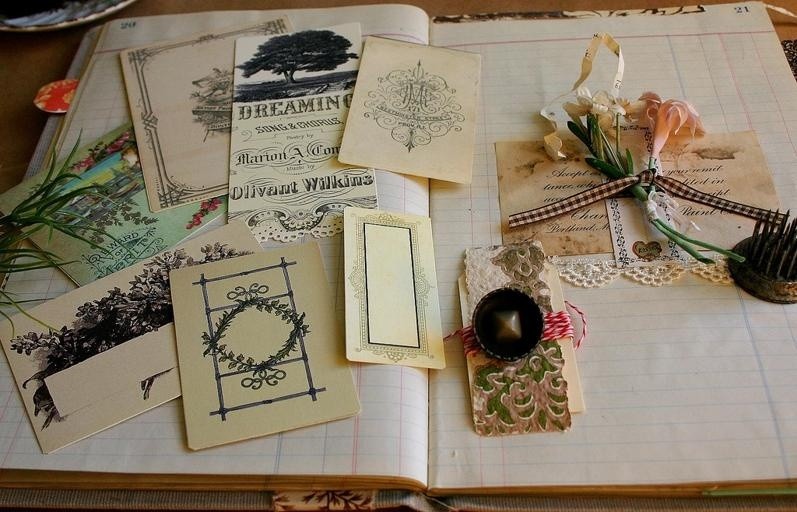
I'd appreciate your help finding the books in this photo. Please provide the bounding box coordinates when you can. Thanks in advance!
[0,3,797,498]
